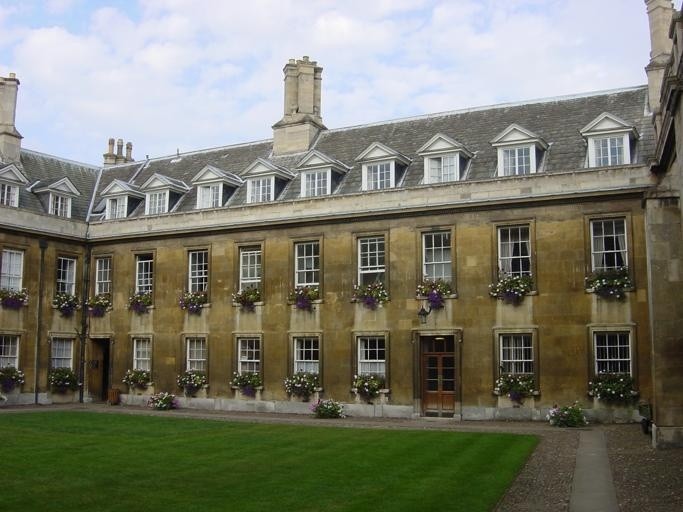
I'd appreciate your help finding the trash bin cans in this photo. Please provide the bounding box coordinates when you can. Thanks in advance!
[108,389,120,405]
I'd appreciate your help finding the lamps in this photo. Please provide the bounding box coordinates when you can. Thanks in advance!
[417,305,431,326]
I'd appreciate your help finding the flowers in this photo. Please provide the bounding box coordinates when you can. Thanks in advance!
[178,289,208,316]
[308,400,350,419]
[147,392,179,411]
[543,400,590,425]
[349,279,389,312]
[122,368,151,392]
[231,284,262,312]
[285,369,323,400]
[1,360,25,393]
[176,366,208,396]
[416,279,452,309]
[352,371,386,401]
[51,292,83,318]
[85,291,112,317]
[585,268,632,303]
[485,271,534,304]
[587,369,637,409]
[2,286,28,309]
[125,290,153,316]
[286,284,320,313]
[229,368,263,399]
[493,371,545,405]
[49,365,79,394]
